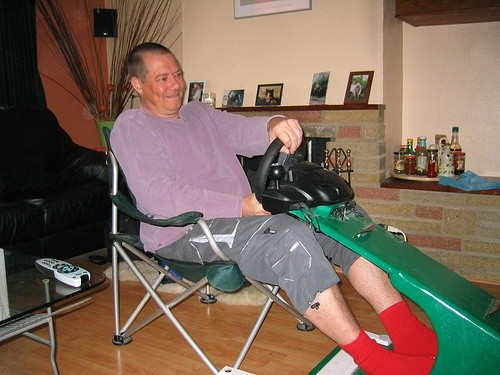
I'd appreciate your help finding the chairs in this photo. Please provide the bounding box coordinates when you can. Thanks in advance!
[102,125,313,375]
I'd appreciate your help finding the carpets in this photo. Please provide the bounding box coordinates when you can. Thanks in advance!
[103,261,270,306]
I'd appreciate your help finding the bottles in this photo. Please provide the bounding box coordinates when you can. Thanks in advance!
[416,137,427,175]
[449,127,462,152]
[403,139,416,157]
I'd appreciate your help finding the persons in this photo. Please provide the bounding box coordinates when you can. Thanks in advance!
[193,83,202,102]
[108,42,439,375]
[352,82,362,99]
[191,83,197,96]
[349,81,356,97]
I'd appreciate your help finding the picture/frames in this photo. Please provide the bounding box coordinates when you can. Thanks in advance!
[233,1,313,19]
[185,80,206,103]
[255,83,283,106]
[344,71,375,104]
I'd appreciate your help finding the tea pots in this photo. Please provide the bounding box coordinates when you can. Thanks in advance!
[436,137,456,177]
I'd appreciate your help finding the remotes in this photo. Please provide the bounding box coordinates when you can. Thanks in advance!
[34,258,91,288]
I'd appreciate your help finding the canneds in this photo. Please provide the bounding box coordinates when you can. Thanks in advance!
[393,152,406,175]
[453,152,466,176]
[416,145,427,175]
[426,149,439,178]
[404,156,415,176]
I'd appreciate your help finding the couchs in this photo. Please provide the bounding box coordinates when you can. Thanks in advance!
[0,108,139,258]
